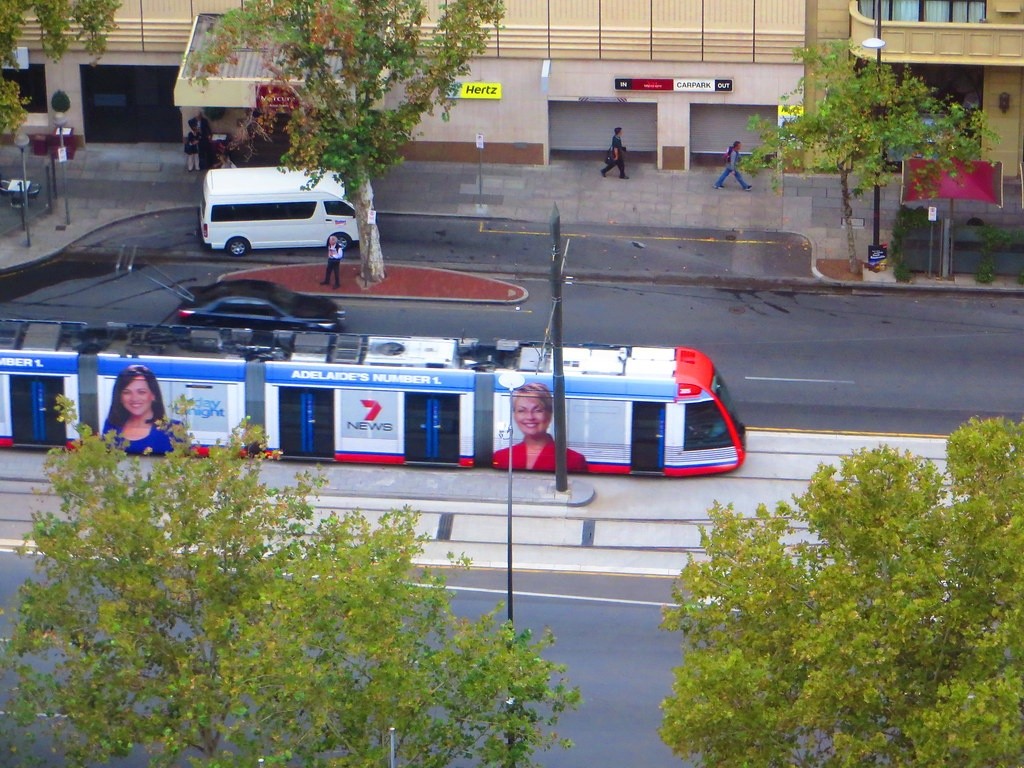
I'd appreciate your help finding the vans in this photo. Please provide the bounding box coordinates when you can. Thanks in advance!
[198,166,358,256]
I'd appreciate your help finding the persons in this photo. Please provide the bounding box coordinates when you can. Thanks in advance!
[183,110,237,170]
[712,140,752,190]
[100,364,185,455]
[319,237,343,289]
[494,383,588,475]
[601,127,630,179]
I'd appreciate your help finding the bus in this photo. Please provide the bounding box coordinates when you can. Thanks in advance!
[0,314,748,477]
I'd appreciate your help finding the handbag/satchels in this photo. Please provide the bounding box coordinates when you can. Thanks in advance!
[605,152,614,164]
[185,143,193,155]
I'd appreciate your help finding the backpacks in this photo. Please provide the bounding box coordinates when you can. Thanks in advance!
[722,146,738,163]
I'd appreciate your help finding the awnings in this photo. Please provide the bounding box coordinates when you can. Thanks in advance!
[173,13,389,112]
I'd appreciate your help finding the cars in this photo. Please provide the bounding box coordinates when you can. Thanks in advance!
[176,278,347,332]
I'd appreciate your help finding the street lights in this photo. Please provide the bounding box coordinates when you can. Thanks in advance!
[15,132,30,247]
[53,112,72,227]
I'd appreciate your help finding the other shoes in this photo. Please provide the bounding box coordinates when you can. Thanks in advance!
[334,281,338,288]
[600,170,606,177]
[714,185,725,189]
[320,280,329,284]
[744,186,753,190]
[620,176,629,178]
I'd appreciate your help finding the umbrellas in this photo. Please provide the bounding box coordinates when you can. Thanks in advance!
[900,155,1004,280]
